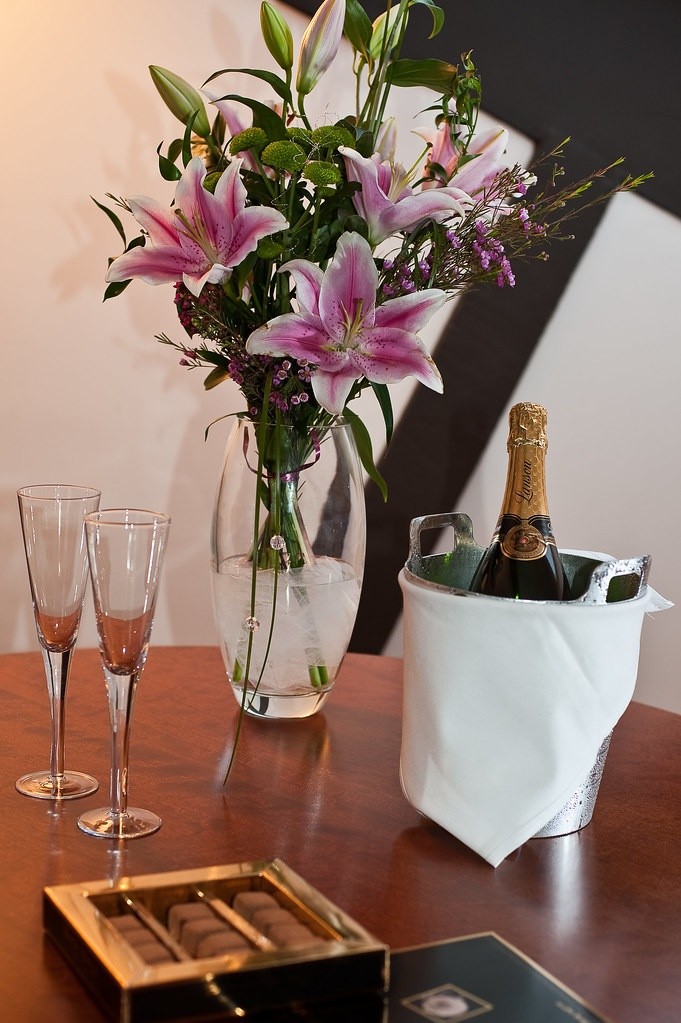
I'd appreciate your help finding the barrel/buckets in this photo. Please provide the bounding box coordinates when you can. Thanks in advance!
[401,510,651,840]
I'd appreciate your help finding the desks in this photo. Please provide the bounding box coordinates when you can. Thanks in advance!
[0,647,681,1023]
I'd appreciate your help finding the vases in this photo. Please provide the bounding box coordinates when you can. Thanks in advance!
[209,410,366,719]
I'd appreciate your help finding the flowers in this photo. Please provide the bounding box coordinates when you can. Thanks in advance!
[87,0,630,505]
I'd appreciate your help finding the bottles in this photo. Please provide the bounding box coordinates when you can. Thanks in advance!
[468,400,575,601]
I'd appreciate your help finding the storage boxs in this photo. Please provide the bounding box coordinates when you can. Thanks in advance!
[42,855,389,1023]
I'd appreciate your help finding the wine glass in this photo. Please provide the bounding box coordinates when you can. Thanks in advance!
[15,484,101,801]
[77,508,172,839]
